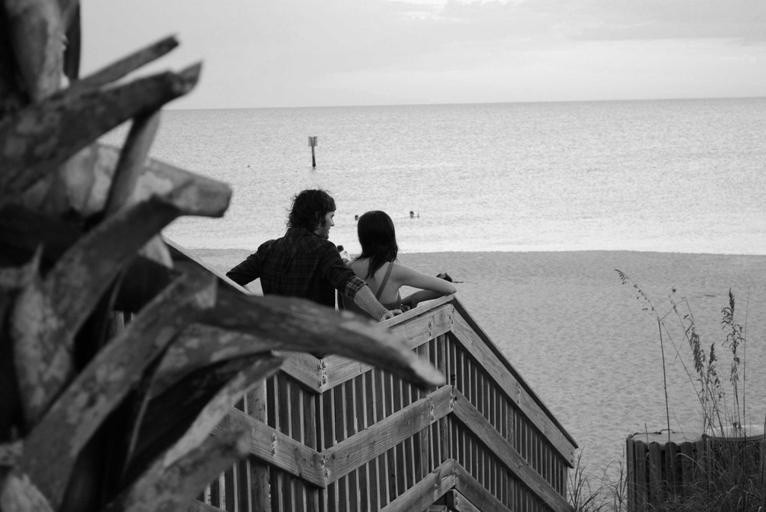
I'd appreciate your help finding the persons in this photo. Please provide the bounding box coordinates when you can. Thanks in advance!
[337,245,351,263]
[337,211,457,319]
[355,215,359,220]
[226,187,403,321]
[410,211,414,218]
